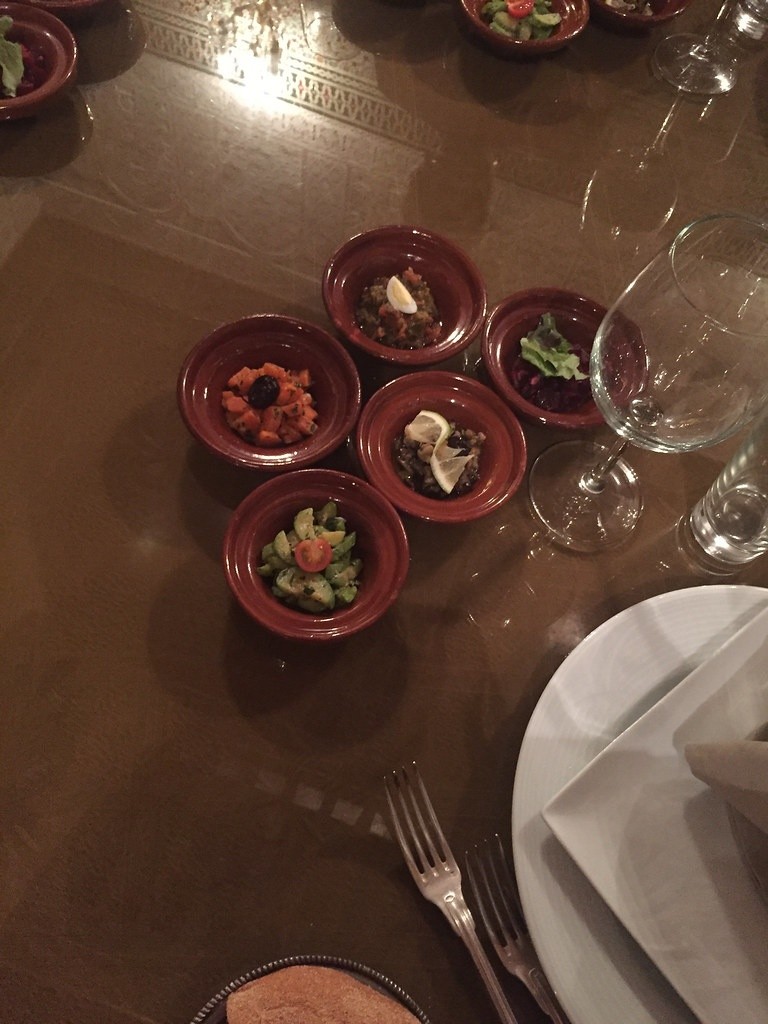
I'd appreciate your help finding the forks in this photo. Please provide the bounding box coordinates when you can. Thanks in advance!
[464,832,571,1024]
[383,762,519,1024]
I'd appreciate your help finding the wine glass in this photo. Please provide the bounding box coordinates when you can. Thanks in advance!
[654,0,742,93]
[524,214,768,550]
[581,71,728,245]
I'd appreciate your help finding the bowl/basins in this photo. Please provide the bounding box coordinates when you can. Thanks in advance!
[176,314,360,471]
[358,371,527,524]
[479,287,646,428]
[225,469,408,641]
[0,3,77,121]
[0,0,100,18]
[461,0,590,59]
[592,0,689,28]
[321,224,486,366]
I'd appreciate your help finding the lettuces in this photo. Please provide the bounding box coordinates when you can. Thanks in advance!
[0,15,25,99]
[519,311,590,381]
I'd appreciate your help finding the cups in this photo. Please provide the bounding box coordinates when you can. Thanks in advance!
[723,0,768,49]
[684,413,768,565]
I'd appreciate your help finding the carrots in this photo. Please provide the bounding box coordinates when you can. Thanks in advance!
[222,361,319,447]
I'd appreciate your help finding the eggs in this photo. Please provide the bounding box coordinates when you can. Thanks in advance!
[387,276,417,314]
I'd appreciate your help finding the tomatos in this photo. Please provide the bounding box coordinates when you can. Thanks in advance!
[294,539,333,572]
[506,0,535,18]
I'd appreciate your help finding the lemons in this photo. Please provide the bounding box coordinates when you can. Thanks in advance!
[406,409,475,494]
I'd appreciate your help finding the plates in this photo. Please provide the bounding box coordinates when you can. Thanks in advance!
[542,606,768,1024]
[513,585,768,1024]
[190,955,430,1024]
